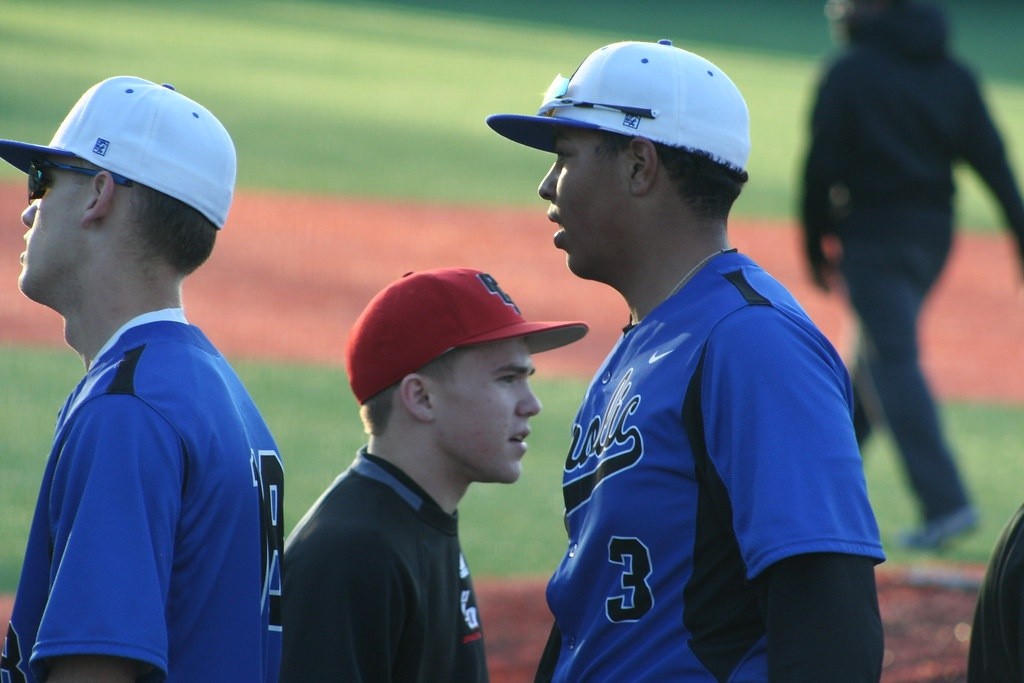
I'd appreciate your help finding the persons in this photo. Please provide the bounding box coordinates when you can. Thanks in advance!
[485,39,886,683]
[278,269,590,683]
[0,76,284,683]
[799,0,1024,556]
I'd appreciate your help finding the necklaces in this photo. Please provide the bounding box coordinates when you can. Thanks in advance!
[664,250,721,301]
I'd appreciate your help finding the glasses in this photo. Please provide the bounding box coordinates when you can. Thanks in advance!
[536,73,657,120]
[28,158,133,192]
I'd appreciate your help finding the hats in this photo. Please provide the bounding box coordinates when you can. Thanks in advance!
[344,267,589,406]
[0,75,237,231]
[485,39,751,182]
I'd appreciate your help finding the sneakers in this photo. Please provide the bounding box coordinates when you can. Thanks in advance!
[895,503,979,553]
